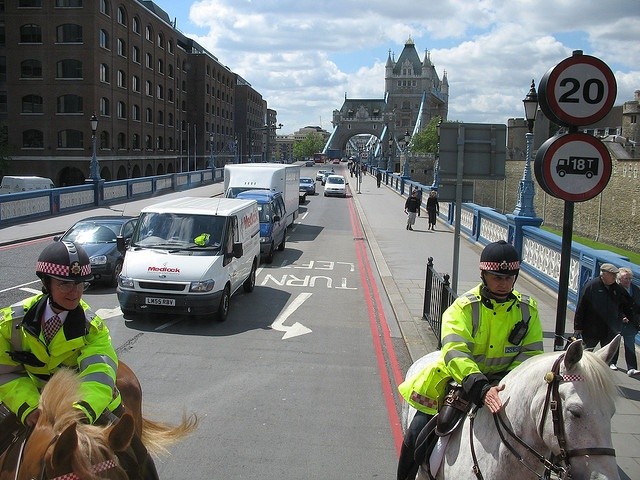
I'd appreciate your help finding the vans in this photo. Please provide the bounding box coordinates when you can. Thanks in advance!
[1,176,57,194]
[235,190,287,263]
[116,196,261,321]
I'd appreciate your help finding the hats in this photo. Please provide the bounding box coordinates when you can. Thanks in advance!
[600,263,620,273]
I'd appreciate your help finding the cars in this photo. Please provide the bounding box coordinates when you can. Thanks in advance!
[316,170,326,180]
[333,159,339,164]
[324,175,349,197]
[299,177,316,195]
[341,157,348,162]
[322,172,336,185]
[53,215,139,288]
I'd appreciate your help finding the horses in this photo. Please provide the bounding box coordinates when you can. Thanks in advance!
[0,360,200,480]
[400,333,622,480]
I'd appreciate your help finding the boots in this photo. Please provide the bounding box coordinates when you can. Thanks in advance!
[397,441,419,480]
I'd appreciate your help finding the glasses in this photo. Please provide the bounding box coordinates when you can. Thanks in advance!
[47,281,92,292]
[621,278,633,281]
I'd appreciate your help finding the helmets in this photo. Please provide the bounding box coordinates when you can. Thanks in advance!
[35,242,93,280]
[480,241,520,277]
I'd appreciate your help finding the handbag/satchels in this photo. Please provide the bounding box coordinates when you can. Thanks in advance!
[563,332,585,349]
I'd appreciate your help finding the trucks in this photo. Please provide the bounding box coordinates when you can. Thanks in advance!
[224,162,300,234]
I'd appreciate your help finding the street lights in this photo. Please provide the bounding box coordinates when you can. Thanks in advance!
[250,139,255,162]
[207,134,215,168]
[234,137,238,163]
[88,112,99,182]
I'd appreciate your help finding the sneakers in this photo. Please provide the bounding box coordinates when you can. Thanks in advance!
[627,368,640,376]
[609,363,617,370]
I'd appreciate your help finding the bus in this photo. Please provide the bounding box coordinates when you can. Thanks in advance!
[314,153,325,163]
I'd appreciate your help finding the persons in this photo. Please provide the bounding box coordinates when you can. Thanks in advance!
[426,190,440,231]
[608,267,640,377]
[376,170,382,188]
[396,238,545,478]
[1,240,158,479]
[349,160,368,178]
[413,185,422,217]
[404,190,421,231]
[573,263,628,352]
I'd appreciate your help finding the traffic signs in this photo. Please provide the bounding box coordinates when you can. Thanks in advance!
[544,54,617,127]
